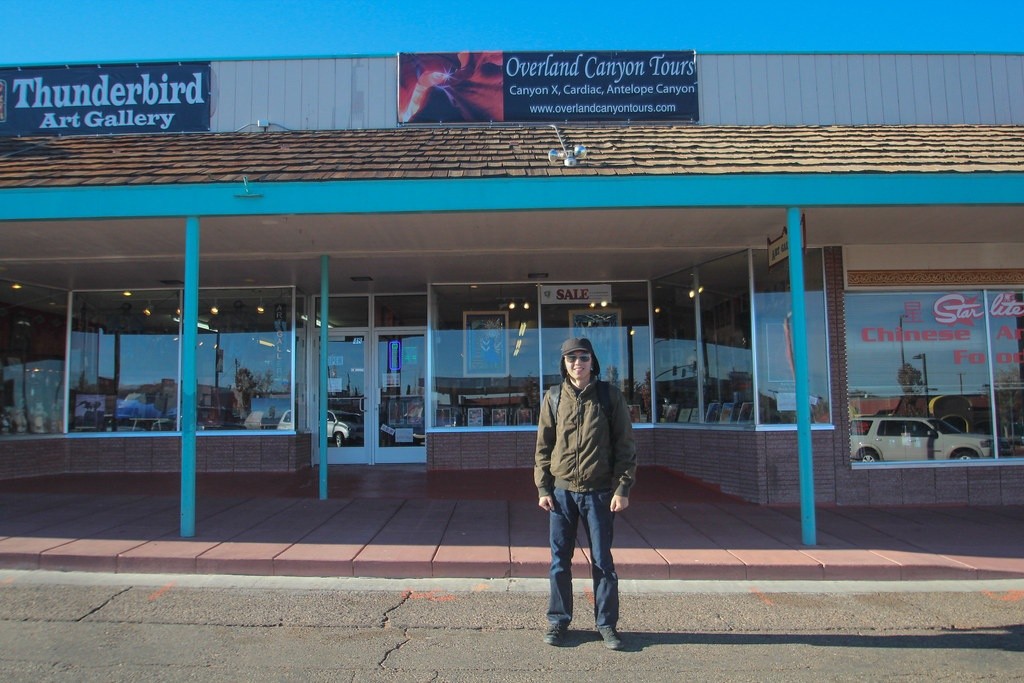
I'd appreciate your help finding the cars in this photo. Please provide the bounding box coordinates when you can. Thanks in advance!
[275,407,364,448]
[171,406,247,430]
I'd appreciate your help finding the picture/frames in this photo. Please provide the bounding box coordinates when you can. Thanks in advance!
[664,402,754,423]
[568,309,625,393]
[628,405,642,424]
[388,401,424,426]
[462,310,510,378]
[435,408,533,427]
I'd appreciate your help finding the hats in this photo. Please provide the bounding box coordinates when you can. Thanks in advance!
[560,338,600,379]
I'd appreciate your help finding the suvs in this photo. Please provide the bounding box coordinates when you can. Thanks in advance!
[850,416,1013,463]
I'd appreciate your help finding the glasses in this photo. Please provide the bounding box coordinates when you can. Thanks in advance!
[567,353,590,362]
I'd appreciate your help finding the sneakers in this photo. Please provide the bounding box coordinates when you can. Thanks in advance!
[544,624,567,645]
[598,628,624,649]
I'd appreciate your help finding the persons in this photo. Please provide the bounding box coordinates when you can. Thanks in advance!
[534,339,638,650]
